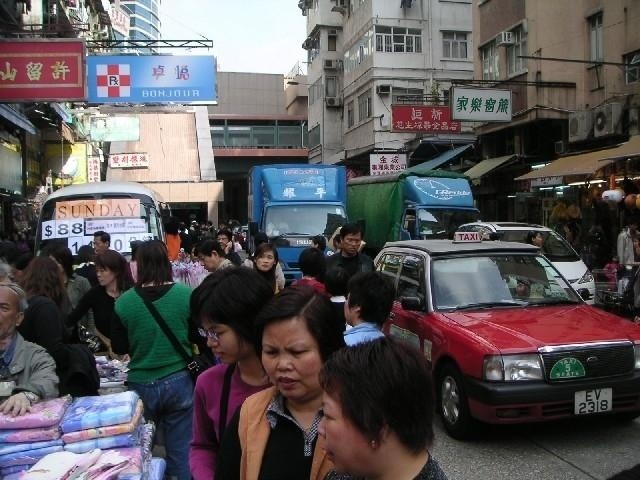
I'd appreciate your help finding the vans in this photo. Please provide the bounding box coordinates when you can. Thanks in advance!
[450,214,598,308]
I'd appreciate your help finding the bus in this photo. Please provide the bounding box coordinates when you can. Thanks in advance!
[28,176,174,270]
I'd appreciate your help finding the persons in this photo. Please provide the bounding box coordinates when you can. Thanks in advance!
[527,231,543,247]
[616,217,640,324]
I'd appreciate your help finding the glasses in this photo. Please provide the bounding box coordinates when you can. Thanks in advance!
[198,328,230,343]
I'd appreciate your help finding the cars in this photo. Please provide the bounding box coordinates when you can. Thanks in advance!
[232,223,248,249]
[360,223,640,442]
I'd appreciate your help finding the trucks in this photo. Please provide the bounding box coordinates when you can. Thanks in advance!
[344,168,485,248]
[242,160,350,282]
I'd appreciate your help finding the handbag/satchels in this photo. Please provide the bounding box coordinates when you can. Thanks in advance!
[135,285,214,384]
